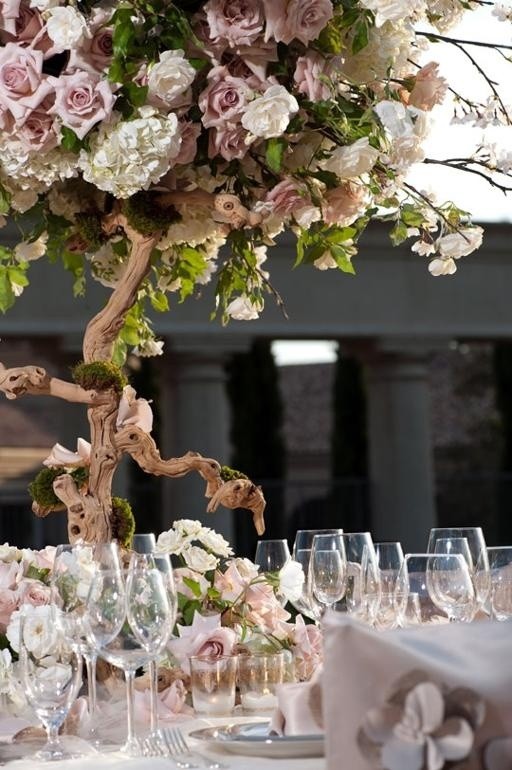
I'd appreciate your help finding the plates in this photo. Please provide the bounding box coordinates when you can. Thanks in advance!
[186,720,327,760]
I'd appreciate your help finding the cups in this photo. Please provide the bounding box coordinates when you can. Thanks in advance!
[188,651,285,720]
[254,526,512,632]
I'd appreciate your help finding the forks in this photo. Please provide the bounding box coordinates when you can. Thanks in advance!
[134,726,223,770]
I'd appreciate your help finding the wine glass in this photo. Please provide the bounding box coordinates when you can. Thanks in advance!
[21,533,181,764]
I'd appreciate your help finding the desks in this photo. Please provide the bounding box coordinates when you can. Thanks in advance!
[0,645,512,770]
